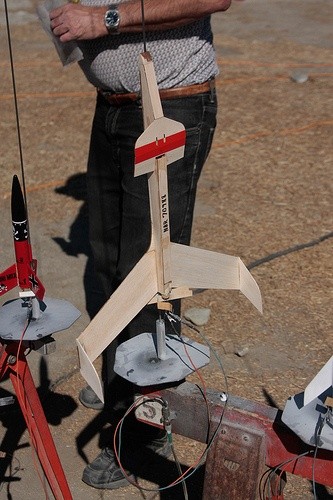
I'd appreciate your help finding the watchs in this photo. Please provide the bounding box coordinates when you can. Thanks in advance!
[105,4,122,36]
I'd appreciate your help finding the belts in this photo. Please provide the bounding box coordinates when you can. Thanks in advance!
[95,74,218,103]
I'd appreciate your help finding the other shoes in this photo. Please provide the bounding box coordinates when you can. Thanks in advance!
[78,385,105,410]
[81,429,173,489]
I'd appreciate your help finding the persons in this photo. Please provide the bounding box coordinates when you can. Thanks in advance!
[48,1,232,488]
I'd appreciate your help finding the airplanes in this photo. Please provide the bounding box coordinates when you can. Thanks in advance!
[75,52,263,404]
